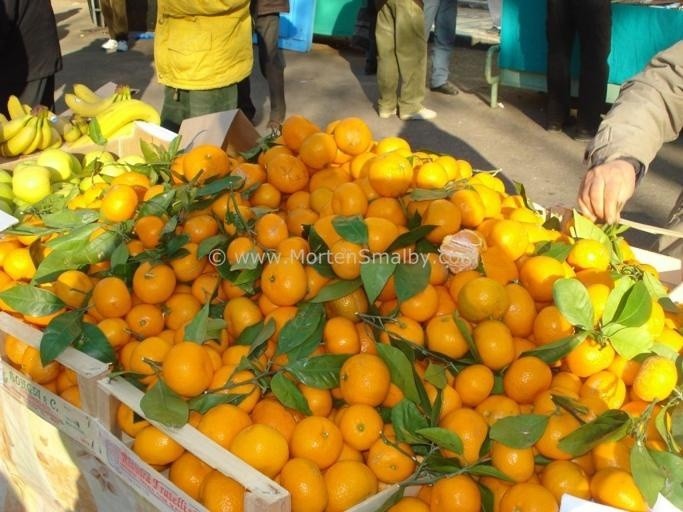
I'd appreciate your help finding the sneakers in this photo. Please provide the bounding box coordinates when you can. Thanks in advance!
[377,102,399,119]
[544,115,577,134]
[101,39,128,54]
[400,106,437,121]
[429,82,459,96]
[571,117,604,143]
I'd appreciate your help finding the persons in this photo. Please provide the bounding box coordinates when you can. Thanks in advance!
[370,0,437,120]
[150,0,254,132]
[544,1,610,134]
[416,1,458,95]
[361,0,379,73]
[578,35,682,281]
[230,1,291,129]
[101,0,129,52]
[0,1,63,117]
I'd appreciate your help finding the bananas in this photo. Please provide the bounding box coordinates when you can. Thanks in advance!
[0,82,162,158]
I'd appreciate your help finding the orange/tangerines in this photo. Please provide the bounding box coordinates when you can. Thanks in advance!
[0,113,683,512]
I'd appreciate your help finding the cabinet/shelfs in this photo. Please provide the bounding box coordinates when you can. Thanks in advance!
[472,1,681,111]
